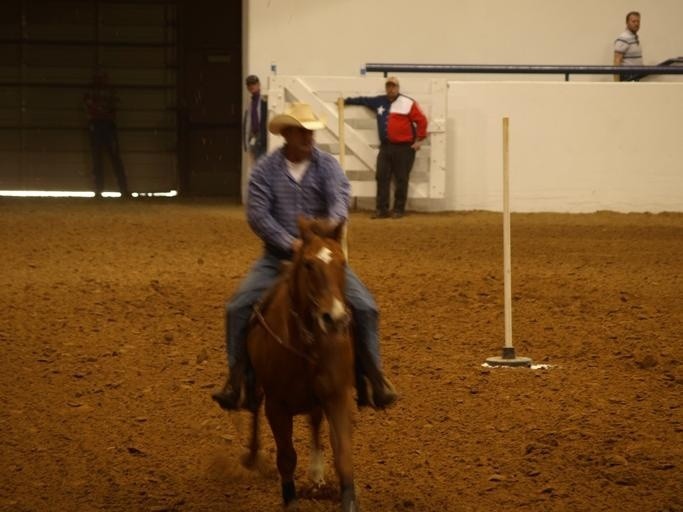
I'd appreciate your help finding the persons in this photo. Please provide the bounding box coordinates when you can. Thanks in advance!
[243,74,267,163]
[332,77,428,220]
[611,11,644,83]
[207,101,396,409]
[81,62,132,200]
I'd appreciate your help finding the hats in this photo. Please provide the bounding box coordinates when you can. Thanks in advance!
[384,76,398,86]
[245,75,259,86]
[266,101,326,134]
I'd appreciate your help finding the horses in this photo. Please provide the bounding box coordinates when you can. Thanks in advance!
[239,213,356,512]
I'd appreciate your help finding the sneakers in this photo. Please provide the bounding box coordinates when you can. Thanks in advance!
[212,386,262,412]
[354,365,397,408]
[370,212,403,218]
